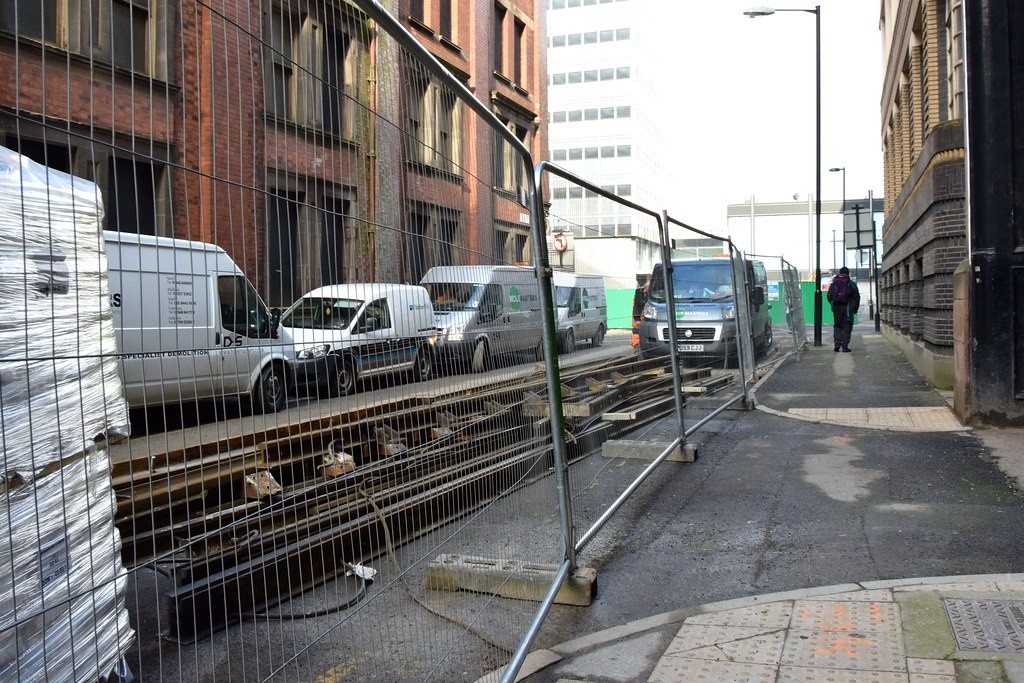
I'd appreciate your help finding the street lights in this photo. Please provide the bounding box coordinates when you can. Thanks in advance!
[830,167,847,268]
[742,3,826,348]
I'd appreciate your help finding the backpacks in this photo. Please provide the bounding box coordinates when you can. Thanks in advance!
[829,274,851,304]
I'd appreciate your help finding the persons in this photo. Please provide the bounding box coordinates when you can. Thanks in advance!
[826,267,861,353]
[631,286,647,347]
[437,286,458,302]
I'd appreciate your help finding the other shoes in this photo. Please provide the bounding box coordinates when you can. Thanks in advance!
[834,348,839,351]
[844,348,851,351]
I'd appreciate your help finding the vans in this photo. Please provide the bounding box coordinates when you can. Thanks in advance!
[737,259,772,354]
[415,264,559,374]
[633,256,750,364]
[276,282,437,397]
[550,269,608,354]
[101,230,300,413]
